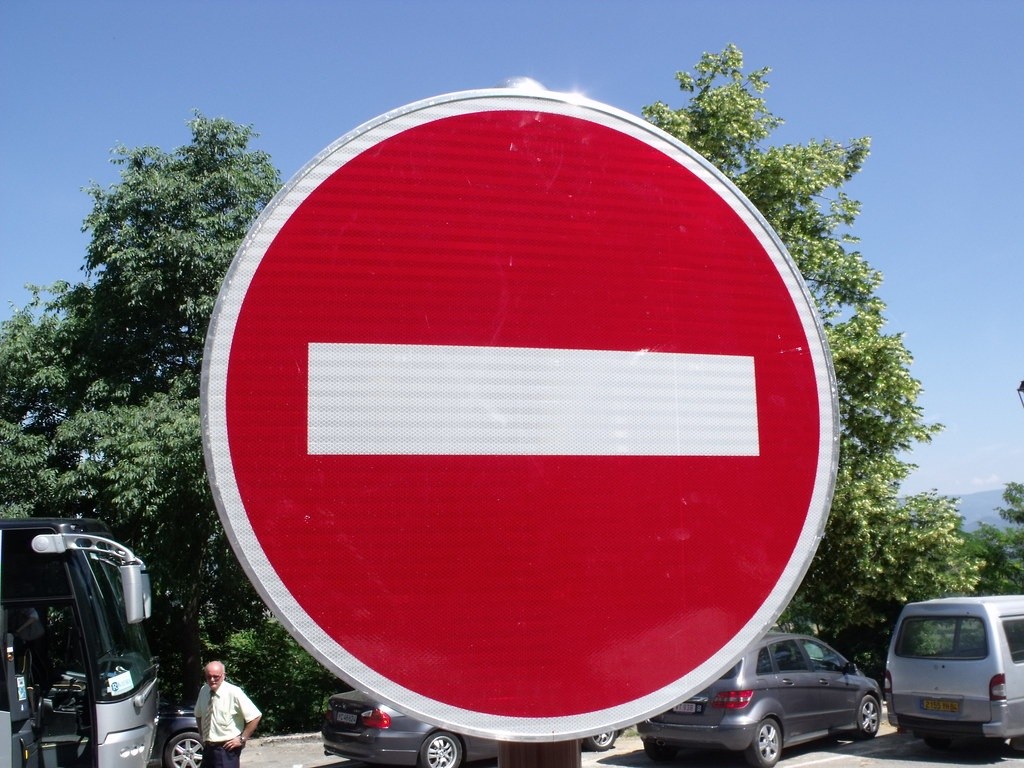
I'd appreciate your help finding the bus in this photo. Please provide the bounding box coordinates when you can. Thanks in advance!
[0,515,158,768]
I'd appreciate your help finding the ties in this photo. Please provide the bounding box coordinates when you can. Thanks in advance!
[203,690,215,739]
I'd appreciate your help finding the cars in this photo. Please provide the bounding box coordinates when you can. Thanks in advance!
[638,631,880,765]
[317,685,626,768]
[151,700,205,768]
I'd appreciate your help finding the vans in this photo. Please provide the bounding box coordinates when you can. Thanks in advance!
[884,592,1023,740]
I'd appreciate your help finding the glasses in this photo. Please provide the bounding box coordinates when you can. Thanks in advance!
[204,673,224,680]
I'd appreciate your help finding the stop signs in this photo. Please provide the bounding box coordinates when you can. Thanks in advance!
[200,80,842,742]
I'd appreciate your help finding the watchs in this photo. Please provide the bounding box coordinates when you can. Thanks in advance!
[240,735,245,744]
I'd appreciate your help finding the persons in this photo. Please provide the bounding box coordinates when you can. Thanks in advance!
[12,607,52,690]
[194,661,262,768]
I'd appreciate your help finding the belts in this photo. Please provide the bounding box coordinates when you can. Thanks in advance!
[205,741,226,747]
